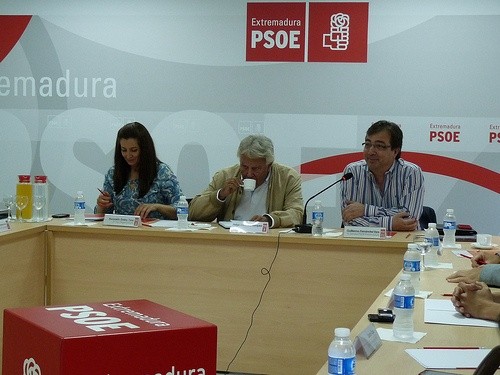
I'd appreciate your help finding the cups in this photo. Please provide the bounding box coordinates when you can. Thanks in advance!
[240,179,256,192]
[478,233,492,245]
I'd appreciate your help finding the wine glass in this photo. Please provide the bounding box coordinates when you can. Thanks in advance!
[2,192,45,223]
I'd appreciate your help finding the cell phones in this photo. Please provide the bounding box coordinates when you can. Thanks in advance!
[52,214,69,218]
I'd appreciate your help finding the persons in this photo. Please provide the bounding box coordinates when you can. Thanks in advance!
[187,134,304,229]
[94,122,185,220]
[446,252,500,322]
[339,120,425,231]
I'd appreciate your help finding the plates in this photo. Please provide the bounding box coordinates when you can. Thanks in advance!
[471,243,498,249]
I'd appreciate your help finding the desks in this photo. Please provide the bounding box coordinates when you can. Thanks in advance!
[0,217,500,374]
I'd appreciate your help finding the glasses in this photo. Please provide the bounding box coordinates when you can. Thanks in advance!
[362,142,395,151]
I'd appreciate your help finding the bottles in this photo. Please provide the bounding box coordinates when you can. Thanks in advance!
[402,208,456,295]
[328,327,356,375]
[177,196,189,229]
[392,274,417,340]
[74,191,85,225]
[312,200,324,236]
[15,175,49,223]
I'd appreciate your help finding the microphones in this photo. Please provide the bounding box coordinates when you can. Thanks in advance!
[294,173,353,233]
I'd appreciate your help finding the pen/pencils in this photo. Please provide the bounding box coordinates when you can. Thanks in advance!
[417,346,479,349]
[440,293,453,296]
[460,254,487,264]
[406,234,411,238]
[97,187,105,196]
[142,223,152,227]
